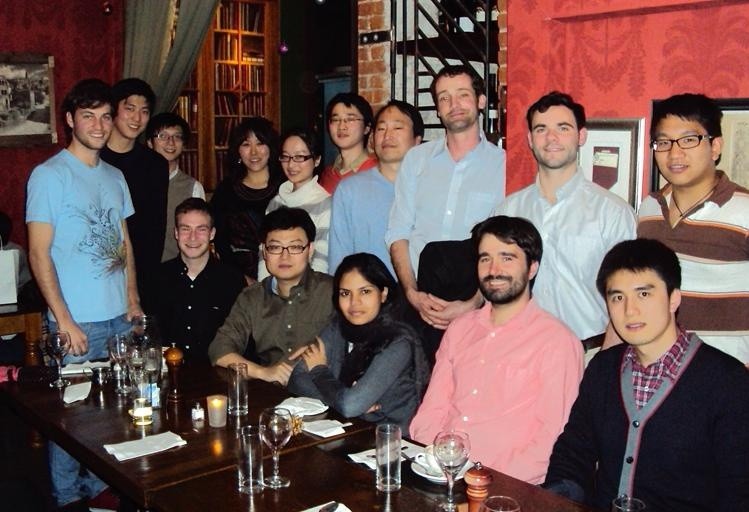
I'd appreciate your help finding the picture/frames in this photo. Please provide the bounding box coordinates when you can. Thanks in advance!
[578,117,645,212]
[651,97,749,193]
[0,52,60,150]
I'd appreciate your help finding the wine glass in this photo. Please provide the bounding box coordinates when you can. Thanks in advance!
[258,408,294,489]
[432,431,471,512]
[44,332,71,389]
[110,334,133,394]
[478,495,522,512]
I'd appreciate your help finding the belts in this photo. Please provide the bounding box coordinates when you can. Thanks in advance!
[581,332,606,354]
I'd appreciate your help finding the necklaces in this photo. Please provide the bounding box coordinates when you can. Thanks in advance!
[669,185,717,218]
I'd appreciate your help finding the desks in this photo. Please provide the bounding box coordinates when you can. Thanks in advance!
[0,335,381,508]
[147,423,600,512]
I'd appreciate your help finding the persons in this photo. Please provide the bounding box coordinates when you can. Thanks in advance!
[326,98,425,284]
[318,92,380,196]
[384,61,506,372]
[144,111,207,279]
[206,203,338,387]
[599,92,749,370]
[407,215,585,491]
[493,87,638,371]
[143,197,248,364]
[207,116,288,287]
[25,77,146,512]
[544,236,748,512]
[0,211,48,366]
[254,128,332,282]
[287,251,433,439]
[100,76,169,311]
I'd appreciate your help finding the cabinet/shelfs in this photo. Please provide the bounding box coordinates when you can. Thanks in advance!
[169,0,282,195]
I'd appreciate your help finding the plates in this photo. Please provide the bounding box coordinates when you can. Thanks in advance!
[410,457,475,484]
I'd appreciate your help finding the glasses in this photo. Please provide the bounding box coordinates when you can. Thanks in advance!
[265,243,309,254]
[279,154,312,162]
[154,132,183,141]
[329,117,365,124]
[650,134,714,152]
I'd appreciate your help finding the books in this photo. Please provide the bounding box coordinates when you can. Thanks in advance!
[170,0,266,201]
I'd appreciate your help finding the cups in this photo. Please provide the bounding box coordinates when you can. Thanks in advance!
[83,366,113,386]
[132,397,153,426]
[237,426,265,493]
[612,498,647,512]
[415,445,442,476]
[374,424,402,492]
[206,395,227,428]
[227,363,248,416]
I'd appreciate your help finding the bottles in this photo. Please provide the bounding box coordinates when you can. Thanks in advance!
[129,334,161,393]
